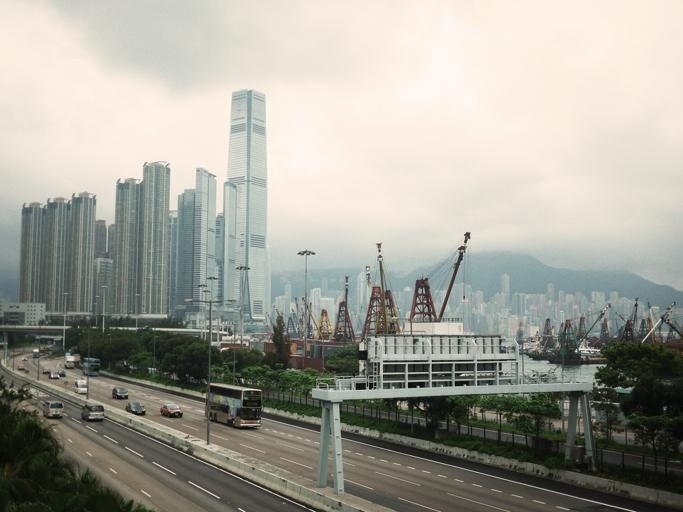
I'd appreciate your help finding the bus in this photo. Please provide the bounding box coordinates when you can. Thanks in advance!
[203,382,263,428]
[81,356,100,377]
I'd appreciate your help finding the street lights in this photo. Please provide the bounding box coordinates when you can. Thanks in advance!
[183,276,240,384]
[93,285,106,335]
[62,293,68,350]
[297,248,316,364]
[235,265,251,345]
[134,293,140,332]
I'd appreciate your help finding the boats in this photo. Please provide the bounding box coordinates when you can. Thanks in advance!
[515,296,683,369]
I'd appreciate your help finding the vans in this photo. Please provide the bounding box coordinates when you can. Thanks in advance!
[42,401,64,419]
[80,403,105,422]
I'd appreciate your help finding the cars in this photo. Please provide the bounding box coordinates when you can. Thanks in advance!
[111,386,128,399]
[159,403,183,418]
[17,346,87,396]
[125,401,145,415]
